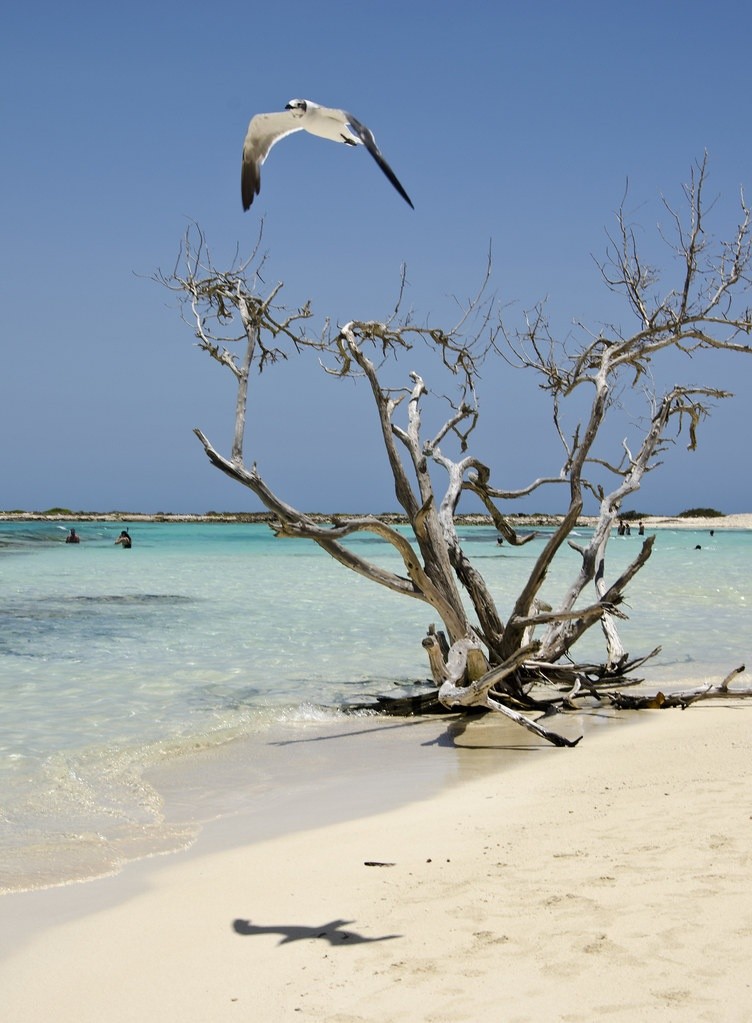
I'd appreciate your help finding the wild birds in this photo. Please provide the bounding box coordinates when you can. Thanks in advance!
[239,95,419,218]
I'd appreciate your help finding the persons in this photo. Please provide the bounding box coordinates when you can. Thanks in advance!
[495,537,504,546]
[617,520,625,535]
[114,531,132,549]
[638,521,645,535]
[625,523,631,535]
[65,529,80,544]
[694,544,702,549]
[710,529,715,536]
